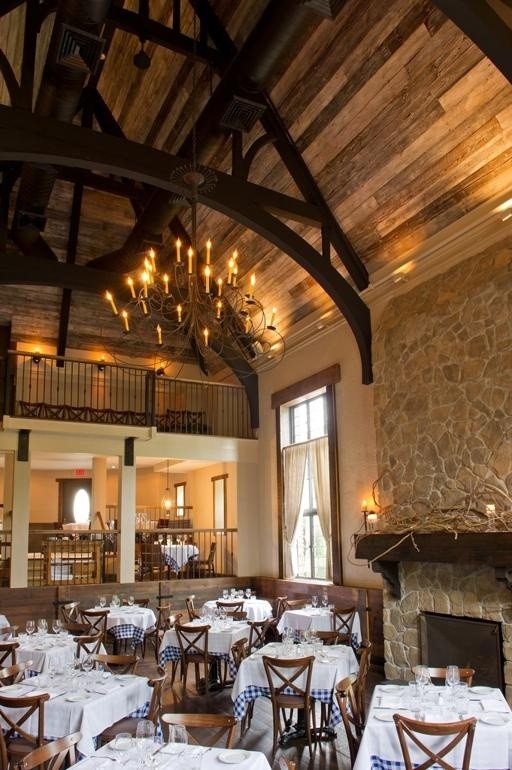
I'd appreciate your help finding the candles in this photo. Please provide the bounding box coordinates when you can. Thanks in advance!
[360,502,368,511]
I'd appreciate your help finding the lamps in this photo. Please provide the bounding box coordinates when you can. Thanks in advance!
[104,163,285,384]
[161,460,173,517]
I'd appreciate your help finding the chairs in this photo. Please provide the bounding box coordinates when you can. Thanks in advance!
[100,667,168,747]
[103,540,217,582]
[335,674,360,770]
[262,654,317,760]
[393,714,477,770]
[160,713,238,748]
[0,590,372,692]
[411,666,475,686]
[0,693,50,770]
[18,730,82,770]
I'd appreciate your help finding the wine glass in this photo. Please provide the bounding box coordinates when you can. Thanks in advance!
[107,719,191,770]
[408,663,471,715]
[39,655,112,692]
[305,594,336,614]
[99,593,137,614]
[154,532,190,548]
[202,613,236,630]
[218,587,257,603]
[277,627,326,660]
[17,617,72,653]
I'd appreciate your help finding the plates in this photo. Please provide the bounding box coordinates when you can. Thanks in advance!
[115,673,141,683]
[67,694,87,706]
[216,747,253,765]
